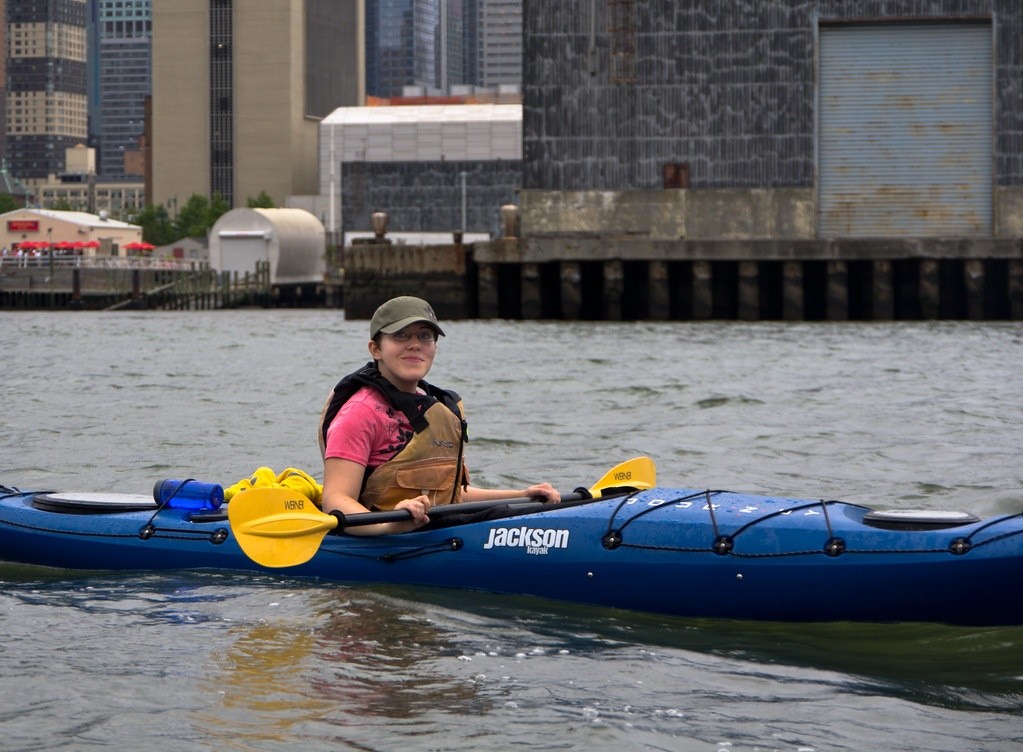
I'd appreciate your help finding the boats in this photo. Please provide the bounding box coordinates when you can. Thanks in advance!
[0,454,1023,628]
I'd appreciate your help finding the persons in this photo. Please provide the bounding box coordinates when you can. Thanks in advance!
[2,247,81,256]
[321,295,561,538]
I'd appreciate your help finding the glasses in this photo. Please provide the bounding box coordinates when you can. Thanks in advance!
[380,329,437,341]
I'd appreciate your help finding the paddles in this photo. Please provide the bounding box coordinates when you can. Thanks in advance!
[227,456,656,568]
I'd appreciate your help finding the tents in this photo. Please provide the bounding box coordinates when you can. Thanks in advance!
[16,241,98,248]
[123,241,157,256]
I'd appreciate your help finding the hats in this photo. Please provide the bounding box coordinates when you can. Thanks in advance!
[370,295,446,340]
